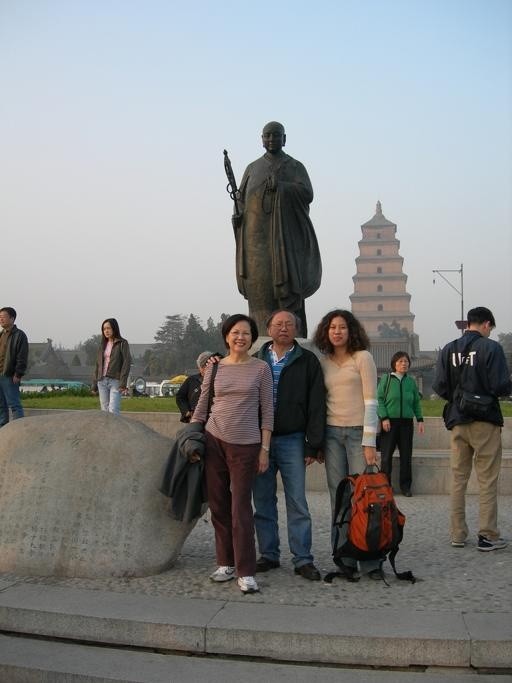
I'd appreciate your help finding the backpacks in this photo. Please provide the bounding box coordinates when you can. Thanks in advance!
[324,463,416,587]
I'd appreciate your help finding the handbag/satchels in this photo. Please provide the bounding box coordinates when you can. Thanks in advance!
[458,392,496,420]
[376,433,396,452]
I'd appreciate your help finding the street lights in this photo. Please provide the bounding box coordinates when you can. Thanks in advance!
[432,263,468,340]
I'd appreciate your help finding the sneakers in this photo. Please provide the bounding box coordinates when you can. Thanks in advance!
[208,557,280,593]
[476,535,508,552]
[295,562,321,580]
[450,538,465,547]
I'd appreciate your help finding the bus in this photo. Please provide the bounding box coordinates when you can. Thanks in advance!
[18,378,89,394]
[146,375,188,398]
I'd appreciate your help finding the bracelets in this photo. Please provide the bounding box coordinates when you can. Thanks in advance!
[261,444,270,453]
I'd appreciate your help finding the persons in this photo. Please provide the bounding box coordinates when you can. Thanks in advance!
[205,309,329,582]
[1,305,30,428]
[376,349,426,497]
[225,121,322,340]
[175,350,223,432]
[184,312,276,594]
[89,317,132,416]
[431,306,510,552]
[313,309,384,582]
